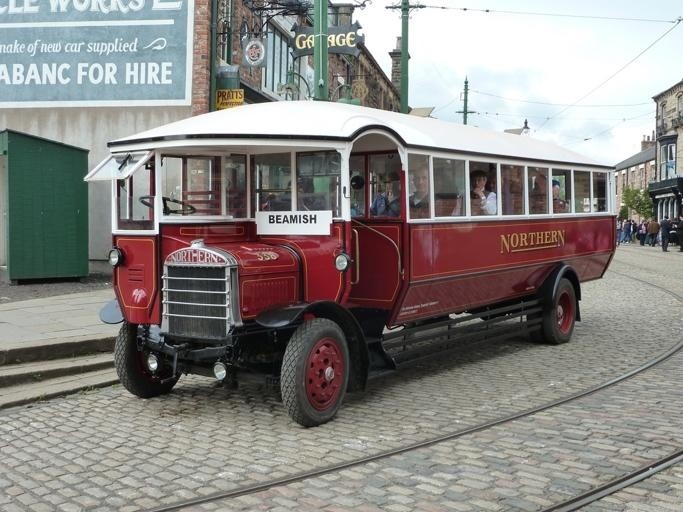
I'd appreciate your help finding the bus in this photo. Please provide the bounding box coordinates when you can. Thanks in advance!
[78,98,622,429]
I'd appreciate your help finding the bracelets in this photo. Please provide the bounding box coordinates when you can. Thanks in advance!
[479,195,487,201]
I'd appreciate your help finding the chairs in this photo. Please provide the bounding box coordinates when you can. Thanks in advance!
[435,193,484,216]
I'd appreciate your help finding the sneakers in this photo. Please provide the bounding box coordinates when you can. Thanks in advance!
[640,242,655,246]
[619,240,632,244]
[663,248,669,252]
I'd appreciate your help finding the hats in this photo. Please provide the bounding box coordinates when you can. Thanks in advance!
[383,172,400,183]
[470,169,484,176]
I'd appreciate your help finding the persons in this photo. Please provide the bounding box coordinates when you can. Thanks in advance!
[408,165,438,219]
[361,172,400,217]
[616,215,682,253]
[448,170,495,218]
[531,175,546,213]
[552,181,569,213]
